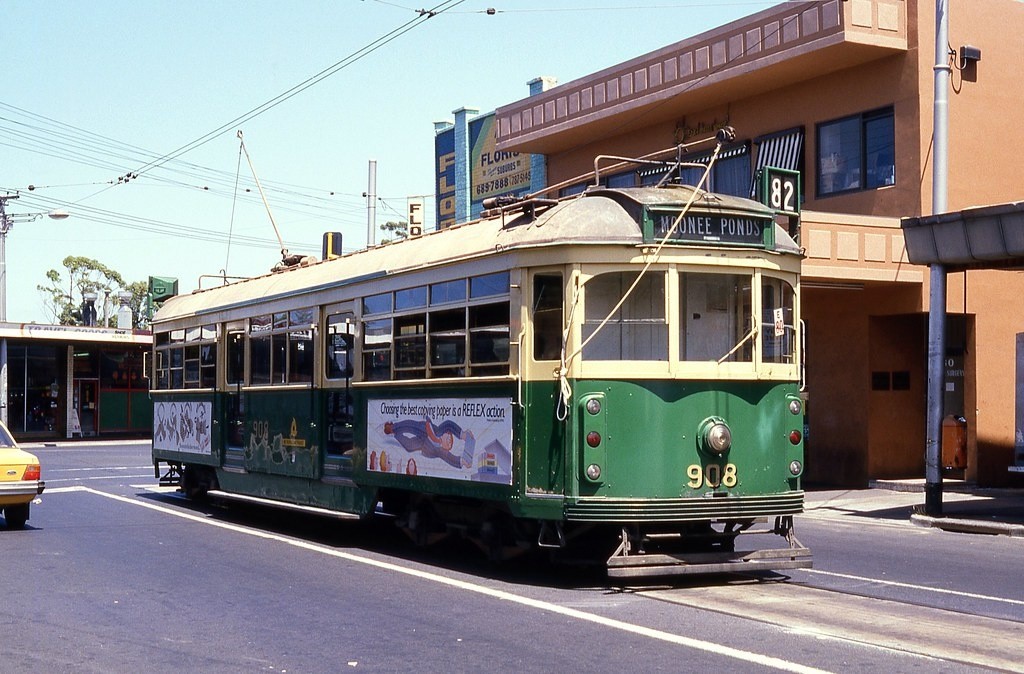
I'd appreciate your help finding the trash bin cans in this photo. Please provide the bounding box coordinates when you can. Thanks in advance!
[941,414,968,472]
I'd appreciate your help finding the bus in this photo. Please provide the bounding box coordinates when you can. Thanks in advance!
[143,126,814,577]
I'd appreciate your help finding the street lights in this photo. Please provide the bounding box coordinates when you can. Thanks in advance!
[0,190,69,426]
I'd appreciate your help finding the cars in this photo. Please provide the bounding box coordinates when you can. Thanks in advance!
[0,420,45,528]
